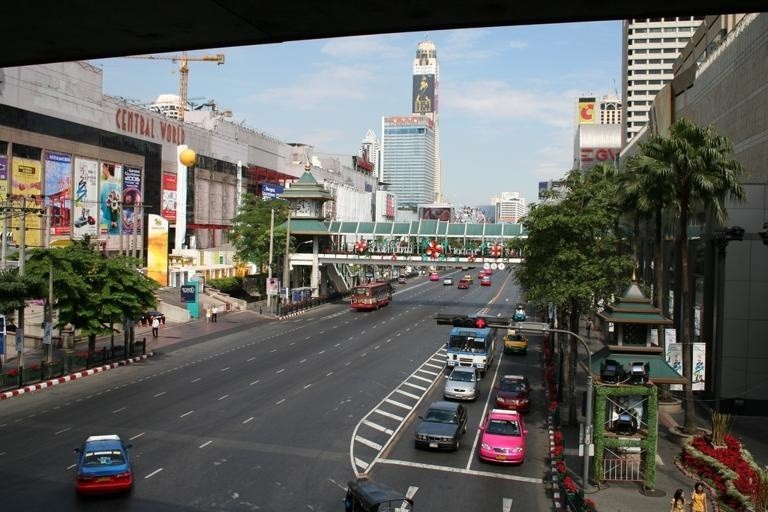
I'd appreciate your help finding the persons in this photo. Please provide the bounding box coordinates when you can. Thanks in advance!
[205,305,212,324]
[99,161,123,234]
[669,487,686,511]
[151,315,159,338]
[689,482,708,511]
[452,206,489,223]
[212,303,218,323]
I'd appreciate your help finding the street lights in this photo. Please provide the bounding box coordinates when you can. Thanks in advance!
[282,240,313,288]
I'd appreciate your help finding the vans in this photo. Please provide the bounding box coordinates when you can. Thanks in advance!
[342,470,415,512]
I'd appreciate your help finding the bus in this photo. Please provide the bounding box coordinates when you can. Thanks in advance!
[351,281,395,311]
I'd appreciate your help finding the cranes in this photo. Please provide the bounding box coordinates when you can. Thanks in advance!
[121,49,225,120]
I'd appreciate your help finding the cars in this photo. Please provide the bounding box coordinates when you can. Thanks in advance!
[74,216,86,228]
[76,430,135,500]
[428,266,492,289]
[415,303,533,468]
[398,276,408,284]
[136,310,166,325]
